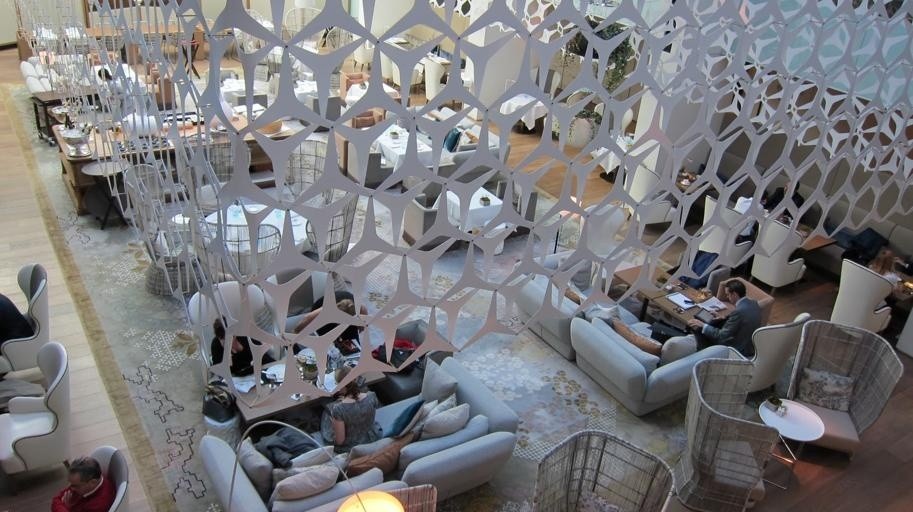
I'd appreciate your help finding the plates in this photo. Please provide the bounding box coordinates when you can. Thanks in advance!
[680,181,690,186]
[265,365,285,382]
[297,348,316,364]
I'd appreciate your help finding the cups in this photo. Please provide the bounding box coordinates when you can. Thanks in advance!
[682,179,688,185]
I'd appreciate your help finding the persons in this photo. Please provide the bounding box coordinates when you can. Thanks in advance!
[0,292,35,355]
[210,315,287,438]
[293,289,368,353]
[686,279,762,352]
[48,454,115,512]
[893,253,912,277]
[865,248,900,312]
[732,184,767,239]
[322,365,383,454]
[95,67,127,113]
[765,179,804,225]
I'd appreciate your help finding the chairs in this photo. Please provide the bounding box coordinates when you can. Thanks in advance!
[0,264,52,371]
[653,154,912,353]
[84,445,129,512]
[530,430,677,512]
[671,358,778,512]
[0,340,76,493]
[771,320,905,465]
[382,483,438,511]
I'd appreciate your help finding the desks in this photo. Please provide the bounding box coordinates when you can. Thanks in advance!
[758,399,825,493]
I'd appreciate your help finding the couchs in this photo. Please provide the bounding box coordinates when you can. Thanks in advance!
[199,356,522,512]
[513,248,773,418]
[0,11,639,291]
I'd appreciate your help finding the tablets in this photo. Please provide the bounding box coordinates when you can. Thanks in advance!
[694,307,714,325]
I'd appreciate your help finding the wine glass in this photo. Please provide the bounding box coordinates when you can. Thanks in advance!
[62,134,92,158]
